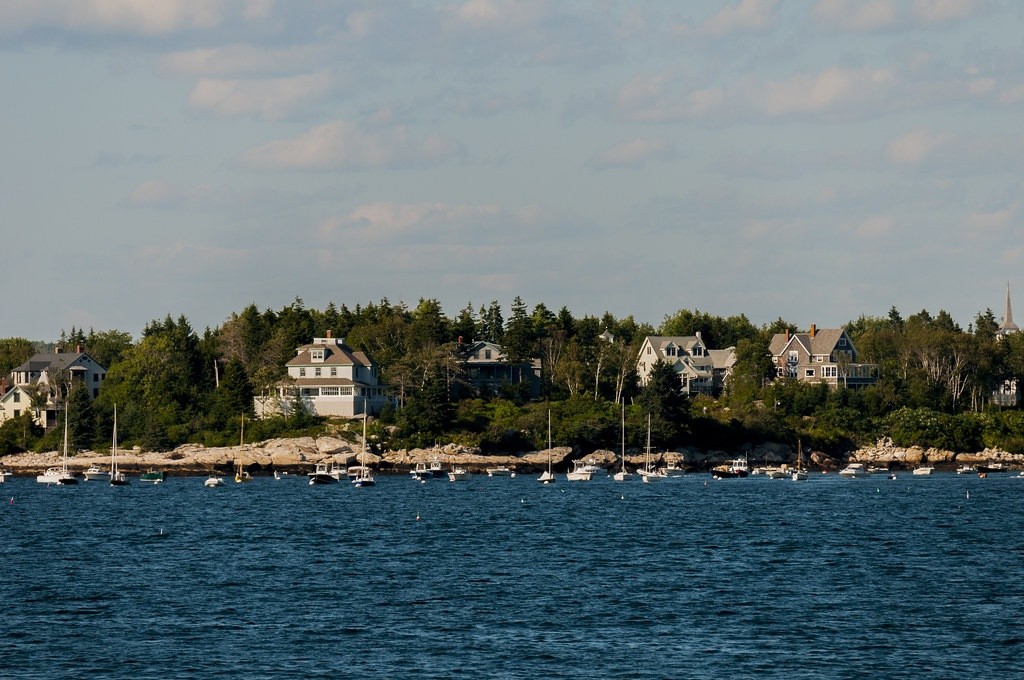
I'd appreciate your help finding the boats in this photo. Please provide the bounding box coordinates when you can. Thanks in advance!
[580,459,607,477]
[409,462,434,480]
[36,466,76,485]
[82,465,112,482]
[566,459,597,481]
[955,463,978,474]
[974,461,1007,473]
[307,454,340,485]
[752,465,807,479]
[659,459,685,476]
[710,459,748,477]
[838,463,871,477]
[427,439,448,478]
[204,474,225,488]
[448,466,469,482]
[1,463,12,483]
[912,462,935,475]
[486,465,516,476]
[273,469,298,479]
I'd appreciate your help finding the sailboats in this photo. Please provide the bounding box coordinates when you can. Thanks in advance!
[613,397,633,481]
[109,402,127,486]
[537,409,557,484]
[792,440,807,481]
[235,411,253,483]
[636,413,667,478]
[59,401,79,485]
[351,399,377,487]
